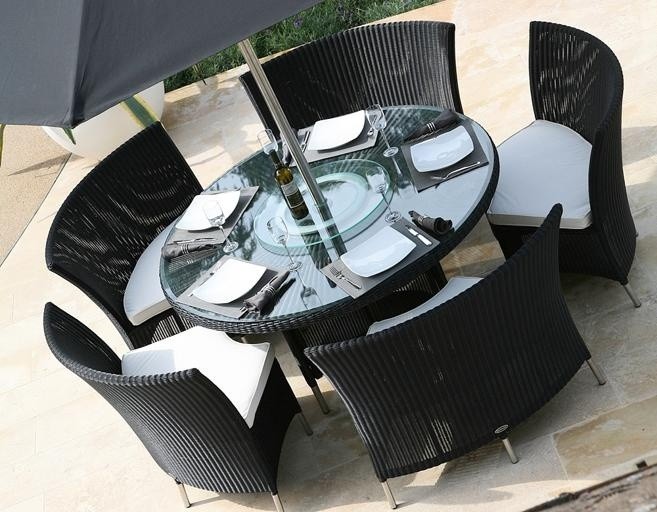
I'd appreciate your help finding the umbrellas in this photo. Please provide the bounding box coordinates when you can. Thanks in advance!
[0,1,327,205]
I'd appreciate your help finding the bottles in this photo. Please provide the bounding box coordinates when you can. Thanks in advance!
[269,149,310,221]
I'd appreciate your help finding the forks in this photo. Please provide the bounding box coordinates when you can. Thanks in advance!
[430,160,481,183]
[328,265,364,291]
[188,260,222,298]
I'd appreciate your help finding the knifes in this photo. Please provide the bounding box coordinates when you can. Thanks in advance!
[298,131,309,155]
[402,222,434,250]
[173,237,216,245]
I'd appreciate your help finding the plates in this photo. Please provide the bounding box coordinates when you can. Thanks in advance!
[192,259,267,306]
[310,110,365,150]
[338,225,414,277]
[174,192,241,229]
[409,125,475,174]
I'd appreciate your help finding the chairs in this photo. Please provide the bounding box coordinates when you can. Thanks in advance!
[482,20,639,309]
[240,21,465,144]
[304,204,606,509]
[42,302,314,512]
[46,122,203,350]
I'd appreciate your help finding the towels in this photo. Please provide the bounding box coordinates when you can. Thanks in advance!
[162,240,217,261]
[404,108,463,143]
[279,129,298,166]
[407,210,453,236]
[244,270,291,314]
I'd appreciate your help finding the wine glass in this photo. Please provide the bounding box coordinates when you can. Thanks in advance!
[256,128,279,159]
[200,199,238,254]
[265,216,302,272]
[364,167,401,224]
[364,103,401,158]
